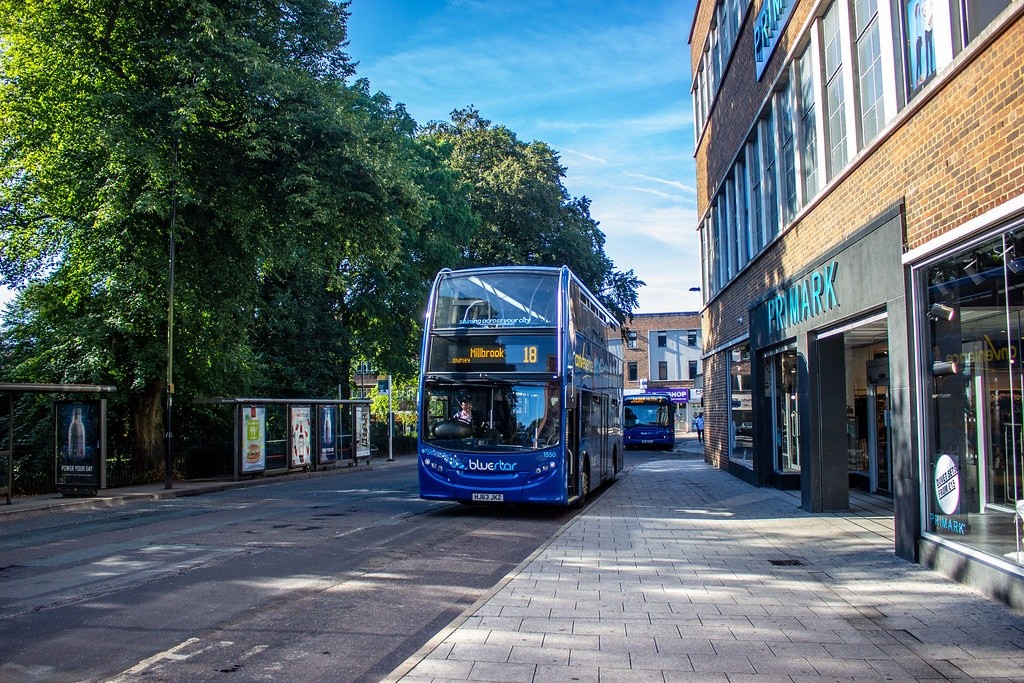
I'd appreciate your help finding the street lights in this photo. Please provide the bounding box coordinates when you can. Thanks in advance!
[162,134,177,492]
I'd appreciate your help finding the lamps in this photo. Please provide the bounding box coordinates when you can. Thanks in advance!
[998,251,1024,274]
[933,360,958,376]
[933,272,950,296]
[962,261,984,286]
[930,302,954,322]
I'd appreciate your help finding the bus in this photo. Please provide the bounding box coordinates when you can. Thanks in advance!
[414,264,626,512]
[622,393,677,451]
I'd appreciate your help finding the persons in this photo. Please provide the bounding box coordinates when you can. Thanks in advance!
[693,412,705,445]
[453,395,474,422]
